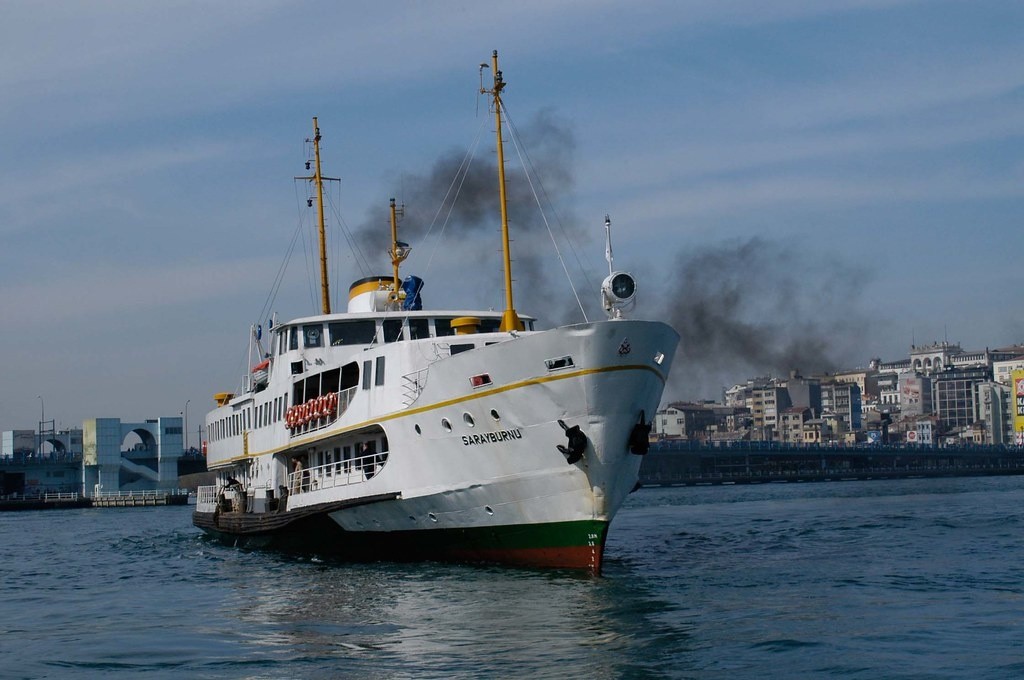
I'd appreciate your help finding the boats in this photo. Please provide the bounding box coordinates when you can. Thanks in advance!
[251,360,269,386]
[192,48,681,577]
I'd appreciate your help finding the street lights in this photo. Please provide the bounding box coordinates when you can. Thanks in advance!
[36,395,44,455]
[185,399,190,452]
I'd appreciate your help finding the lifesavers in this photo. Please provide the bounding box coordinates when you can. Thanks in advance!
[315,396,323,418]
[202,439,207,455]
[291,406,298,428]
[324,392,337,416]
[306,399,314,422]
[298,403,305,425]
[285,407,292,429]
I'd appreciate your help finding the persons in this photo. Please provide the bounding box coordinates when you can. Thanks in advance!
[291,457,303,494]
[222,476,242,505]
[358,442,385,480]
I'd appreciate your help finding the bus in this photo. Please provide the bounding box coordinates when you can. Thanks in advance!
[658,438,699,451]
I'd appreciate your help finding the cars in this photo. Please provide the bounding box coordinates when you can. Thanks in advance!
[891,441,907,450]
